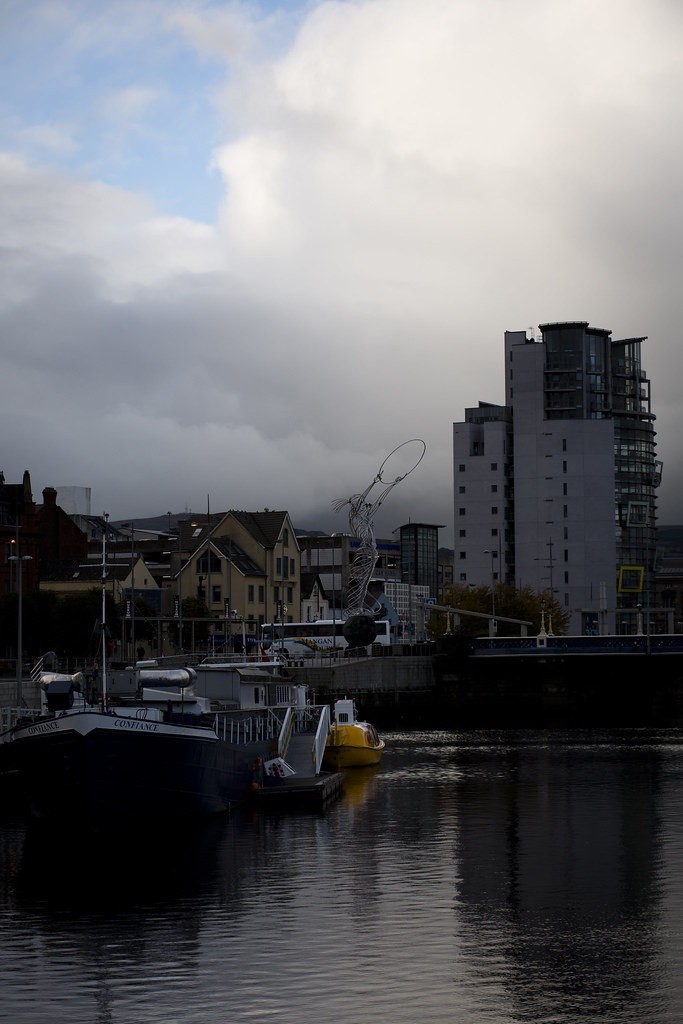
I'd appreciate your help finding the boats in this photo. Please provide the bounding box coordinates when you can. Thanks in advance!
[324,695,385,766]
[0,535,257,827]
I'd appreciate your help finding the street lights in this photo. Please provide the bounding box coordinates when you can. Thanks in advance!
[481,549,496,637]
[7,555,34,707]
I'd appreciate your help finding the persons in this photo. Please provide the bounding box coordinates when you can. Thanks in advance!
[136,644,145,661]
[37,648,58,672]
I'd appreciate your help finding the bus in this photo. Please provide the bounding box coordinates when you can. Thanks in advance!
[260,618,390,659]
